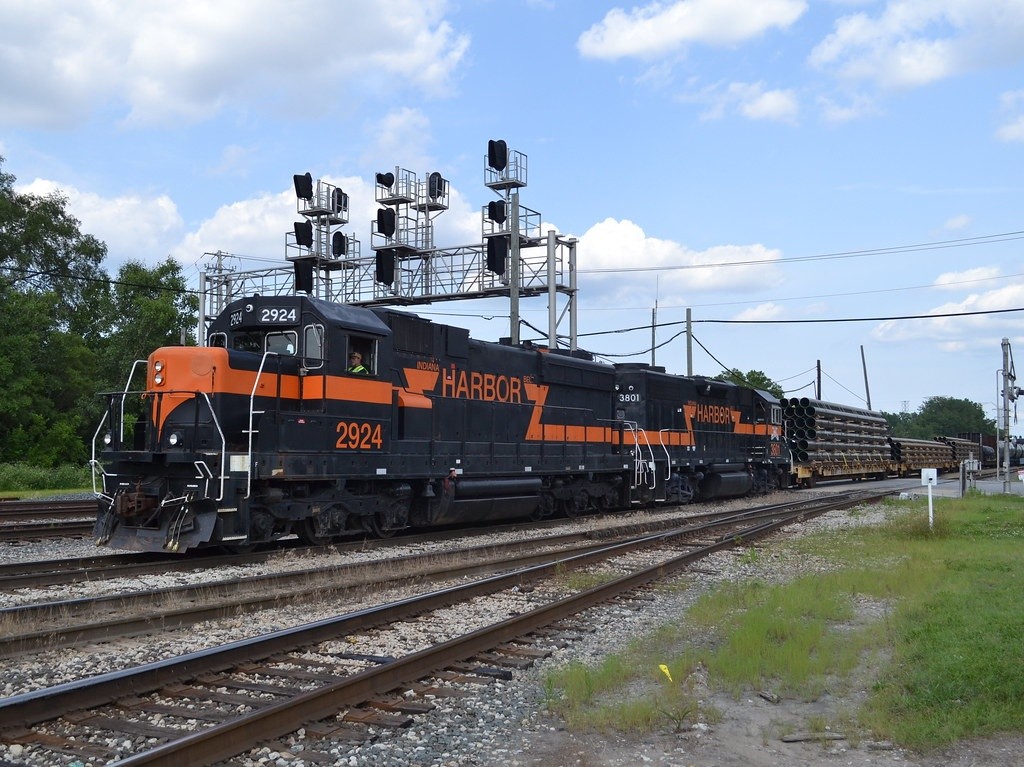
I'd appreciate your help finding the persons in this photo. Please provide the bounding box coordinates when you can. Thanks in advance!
[347,352,369,374]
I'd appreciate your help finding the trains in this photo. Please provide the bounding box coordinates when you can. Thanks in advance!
[89,289,1024,557]
